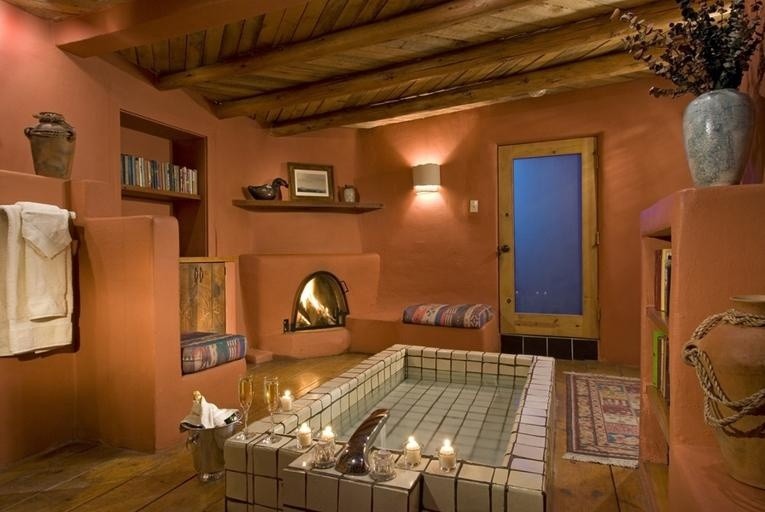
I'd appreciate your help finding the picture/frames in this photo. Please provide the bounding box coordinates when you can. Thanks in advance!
[287,162,337,202]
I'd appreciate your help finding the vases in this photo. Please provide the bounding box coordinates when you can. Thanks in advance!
[682,89,756,185]
[678,295,765,490]
[24,111,77,180]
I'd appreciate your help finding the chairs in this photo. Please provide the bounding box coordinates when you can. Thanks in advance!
[77,217,247,453]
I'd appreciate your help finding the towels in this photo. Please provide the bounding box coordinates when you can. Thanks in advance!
[181,395,240,430]
[0,201,78,357]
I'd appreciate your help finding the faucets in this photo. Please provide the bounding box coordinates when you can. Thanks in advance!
[335,407,391,476]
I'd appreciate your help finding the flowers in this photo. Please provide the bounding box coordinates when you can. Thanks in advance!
[607,0,765,98]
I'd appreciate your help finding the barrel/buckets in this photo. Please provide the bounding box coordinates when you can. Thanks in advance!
[183,416,237,480]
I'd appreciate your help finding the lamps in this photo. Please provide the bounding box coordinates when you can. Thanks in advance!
[412,163,441,192]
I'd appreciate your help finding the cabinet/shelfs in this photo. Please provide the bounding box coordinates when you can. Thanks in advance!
[640,186,765,512]
[179,263,226,332]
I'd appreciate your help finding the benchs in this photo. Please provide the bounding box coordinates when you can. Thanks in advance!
[346,303,498,354]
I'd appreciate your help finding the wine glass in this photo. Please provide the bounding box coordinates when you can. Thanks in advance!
[235,373,256,441]
[260,374,281,443]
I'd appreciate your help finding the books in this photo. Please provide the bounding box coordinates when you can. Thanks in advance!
[646,247,670,405]
[122,151,198,195]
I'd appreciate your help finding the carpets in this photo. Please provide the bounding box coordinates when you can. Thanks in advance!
[562,371,640,469]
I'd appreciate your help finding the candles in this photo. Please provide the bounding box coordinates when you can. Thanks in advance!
[296,422,312,448]
[280,390,293,412]
[320,425,336,441]
[437,438,456,473]
[404,436,421,465]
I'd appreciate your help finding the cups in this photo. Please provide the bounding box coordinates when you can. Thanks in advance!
[281,395,294,411]
[402,440,423,466]
[372,452,394,476]
[312,437,335,468]
[295,429,312,450]
[435,443,459,472]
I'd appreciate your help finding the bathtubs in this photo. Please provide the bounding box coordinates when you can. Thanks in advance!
[224,343,556,511]
